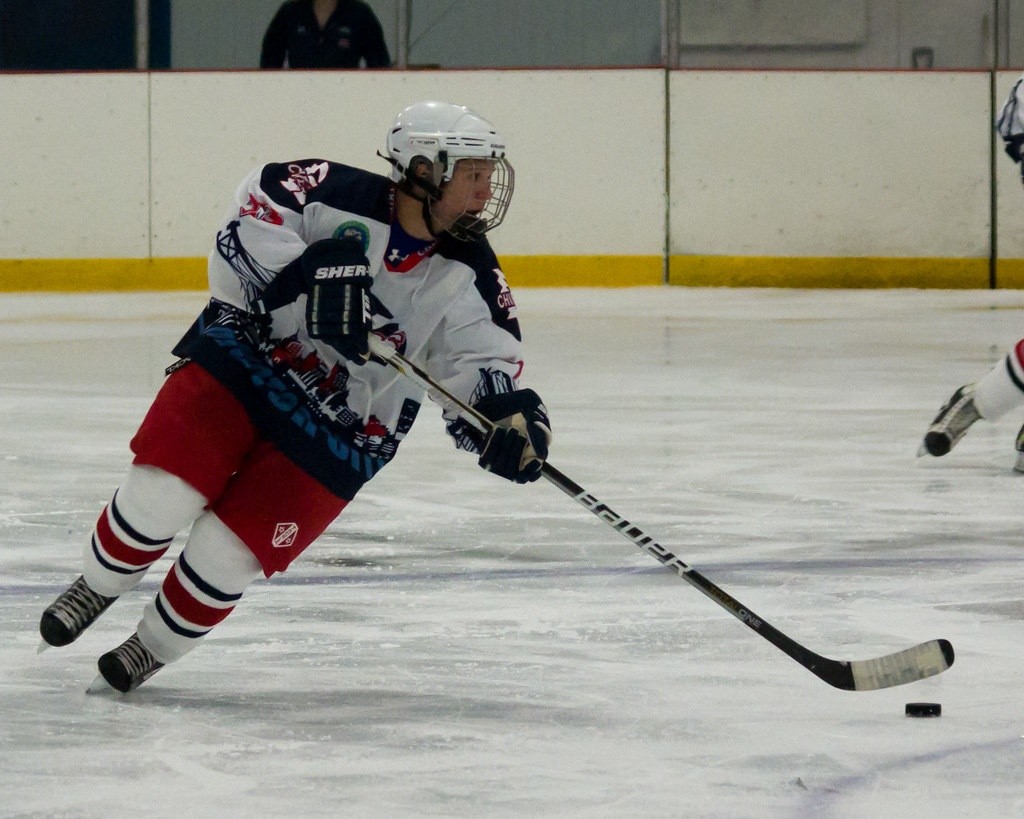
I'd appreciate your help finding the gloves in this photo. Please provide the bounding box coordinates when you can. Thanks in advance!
[463,388,554,484]
[301,232,374,365]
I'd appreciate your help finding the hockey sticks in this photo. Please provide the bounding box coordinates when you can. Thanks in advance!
[369,333,954,690]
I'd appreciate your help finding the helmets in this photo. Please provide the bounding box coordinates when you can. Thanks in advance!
[386,101,506,185]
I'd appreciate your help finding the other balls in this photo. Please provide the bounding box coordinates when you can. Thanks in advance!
[905,703,941,718]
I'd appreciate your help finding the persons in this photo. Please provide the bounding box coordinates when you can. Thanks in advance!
[913,73,1024,473]
[36,99,552,698]
[258,0,392,71]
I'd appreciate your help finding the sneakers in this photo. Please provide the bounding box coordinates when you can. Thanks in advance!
[84,631,166,695]
[916,382,983,457]
[37,574,119,653]
